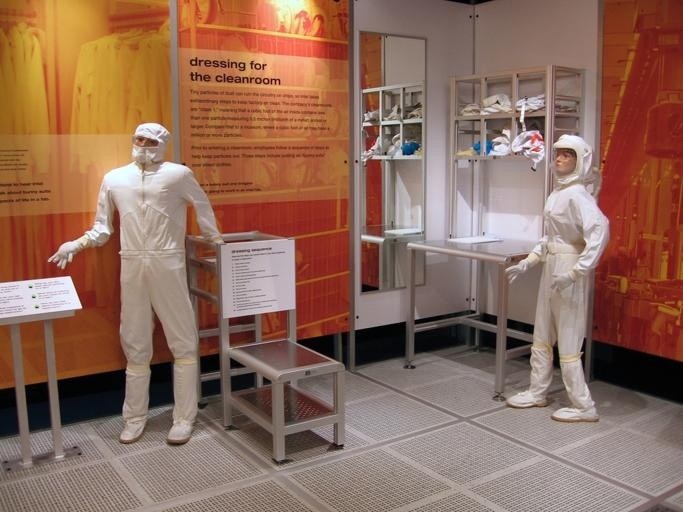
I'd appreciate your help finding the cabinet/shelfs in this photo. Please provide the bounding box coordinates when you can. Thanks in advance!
[404,64,586,402]
[183,227,347,463]
[360,78,427,292]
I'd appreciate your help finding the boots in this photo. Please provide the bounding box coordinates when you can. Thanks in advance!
[120,363,151,443]
[166,359,198,444]
[551,353,599,422]
[506,344,554,409]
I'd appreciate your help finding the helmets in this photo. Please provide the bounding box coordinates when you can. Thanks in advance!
[553,134,593,184]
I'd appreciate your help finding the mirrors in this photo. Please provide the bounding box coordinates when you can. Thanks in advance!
[356,28,430,294]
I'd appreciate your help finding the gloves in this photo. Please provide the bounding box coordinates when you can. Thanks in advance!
[504,252,541,286]
[48,233,92,269]
[550,270,575,295]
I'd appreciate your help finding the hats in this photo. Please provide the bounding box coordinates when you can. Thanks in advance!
[133,123,172,165]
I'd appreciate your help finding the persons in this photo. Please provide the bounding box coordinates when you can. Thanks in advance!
[504,132,609,423]
[46,121,226,446]
